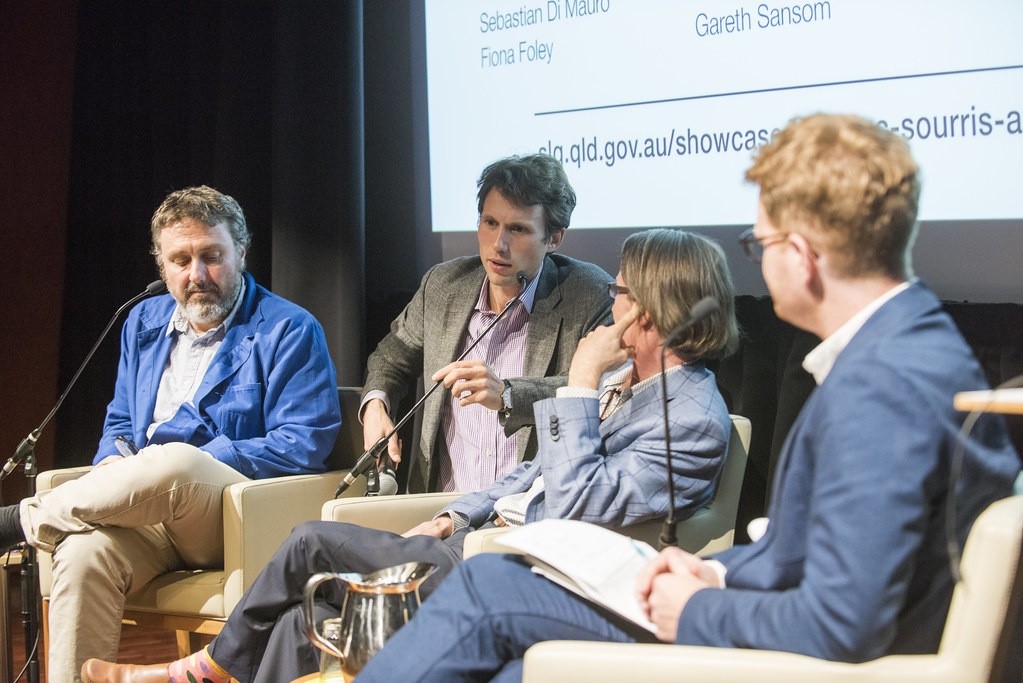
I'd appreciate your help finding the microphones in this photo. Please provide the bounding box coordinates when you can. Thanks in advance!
[341,270,529,486]
[657,295,720,553]
[17,279,165,459]
[377,402,397,497]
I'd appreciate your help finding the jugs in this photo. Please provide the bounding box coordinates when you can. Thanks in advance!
[302,561,442,683]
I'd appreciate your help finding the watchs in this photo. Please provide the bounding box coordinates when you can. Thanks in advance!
[500,379,513,414]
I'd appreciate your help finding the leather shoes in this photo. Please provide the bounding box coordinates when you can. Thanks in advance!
[79,657,173,683]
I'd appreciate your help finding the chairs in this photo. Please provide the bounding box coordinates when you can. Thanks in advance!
[320,412,752,558]
[35,385,395,683]
[520,492,1023,682]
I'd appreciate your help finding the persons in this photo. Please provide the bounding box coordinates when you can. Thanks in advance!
[358,154,618,496]
[350,114,1021,682]
[0,186,342,683]
[79,228,739,683]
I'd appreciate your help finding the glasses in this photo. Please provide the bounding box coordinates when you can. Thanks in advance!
[739,230,818,265]
[608,283,629,299]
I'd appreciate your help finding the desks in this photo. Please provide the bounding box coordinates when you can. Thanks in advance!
[0,549,26,682]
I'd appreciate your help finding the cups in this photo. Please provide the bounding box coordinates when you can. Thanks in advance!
[319,617,345,683]
